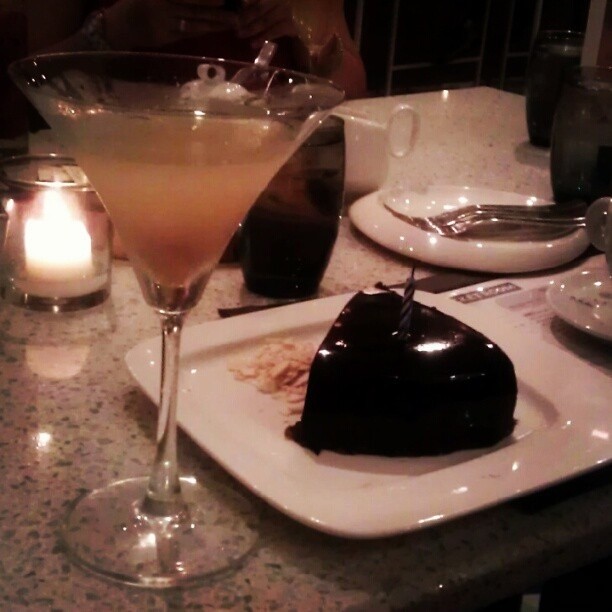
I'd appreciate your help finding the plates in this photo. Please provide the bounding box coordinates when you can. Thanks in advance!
[545,253,612,340]
[123,287,612,543]
[348,183,591,274]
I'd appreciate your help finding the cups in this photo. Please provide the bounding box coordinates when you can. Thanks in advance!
[525,31,585,148]
[549,65,612,218]
[0,156,113,313]
[328,103,423,196]
[240,114,346,300]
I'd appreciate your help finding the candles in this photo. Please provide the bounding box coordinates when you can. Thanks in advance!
[18,174,92,285]
[390,260,426,346]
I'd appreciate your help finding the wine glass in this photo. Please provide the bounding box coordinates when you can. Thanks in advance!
[8,49,347,590]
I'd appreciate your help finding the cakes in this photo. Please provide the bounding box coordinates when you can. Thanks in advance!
[286,277,518,458]
